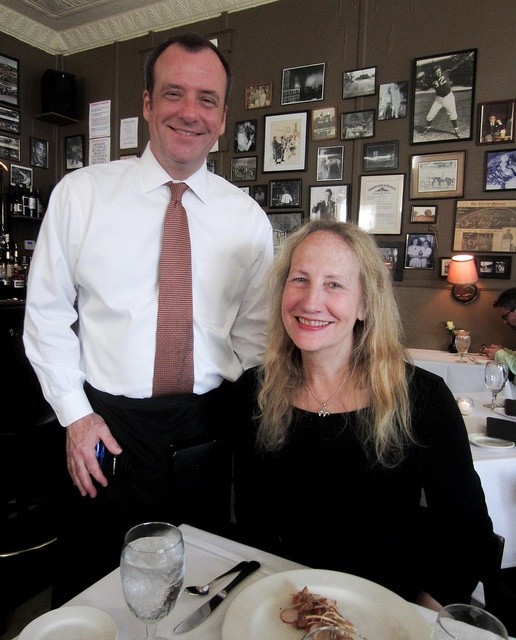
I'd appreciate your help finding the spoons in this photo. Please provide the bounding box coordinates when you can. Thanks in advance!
[184,561,249,597]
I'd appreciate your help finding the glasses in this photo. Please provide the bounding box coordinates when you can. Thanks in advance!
[501,307,515,321]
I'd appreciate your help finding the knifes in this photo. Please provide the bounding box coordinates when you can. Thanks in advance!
[174,562,260,634]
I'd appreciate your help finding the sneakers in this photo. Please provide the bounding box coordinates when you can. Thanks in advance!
[455,128,464,139]
[421,125,431,135]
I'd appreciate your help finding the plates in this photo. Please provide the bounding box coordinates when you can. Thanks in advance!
[18,605,119,639]
[222,569,431,640]
[468,433,515,451]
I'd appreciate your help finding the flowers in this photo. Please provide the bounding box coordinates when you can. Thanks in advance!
[441,320,457,335]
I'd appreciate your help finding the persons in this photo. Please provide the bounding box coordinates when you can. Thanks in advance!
[343,113,368,138]
[484,114,501,142]
[382,87,393,119]
[70,143,79,164]
[255,188,264,200]
[481,287,516,386]
[420,58,467,138]
[281,189,293,205]
[311,189,336,220]
[408,238,421,267]
[421,240,432,267]
[502,228,514,252]
[273,135,295,162]
[22,34,274,608]
[32,140,47,167]
[184,221,495,621]
[488,154,516,189]
[236,121,255,152]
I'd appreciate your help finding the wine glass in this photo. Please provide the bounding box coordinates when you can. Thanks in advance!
[455,335,471,364]
[484,360,509,408]
[120,521,186,639]
[434,602,508,640]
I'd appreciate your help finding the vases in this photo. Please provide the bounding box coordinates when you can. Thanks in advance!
[448,335,459,353]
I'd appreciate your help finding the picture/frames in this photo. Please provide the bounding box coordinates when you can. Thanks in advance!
[0,52,20,108]
[0,134,21,162]
[8,163,34,190]
[376,80,408,122]
[29,136,50,169]
[475,254,513,281]
[118,152,140,160]
[355,172,406,236]
[451,199,516,254]
[341,65,377,100]
[279,62,327,106]
[475,98,516,145]
[265,210,304,255]
[0,105,21,135]
[482,148,516,193]
[233,118,257,153]
[237,185,250,197]
[316,145,346,182]
[362,139,400,172]
[64,134,85,171]
[307,183,353,224]
[268,178,303,209]
[243,80,273,111]
[229,155,257,182]
[408,47,479,144]
[439,256,451,278]
[261,110,311,174]
[310,104,339,142]
[252,184,268,208]
[376,240,406,282]
[408,149,467,200]
[404,232,436,270]
[409,204,438,224]
[338,108,376,142]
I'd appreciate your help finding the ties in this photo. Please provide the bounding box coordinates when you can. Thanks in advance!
[151,181,194,398]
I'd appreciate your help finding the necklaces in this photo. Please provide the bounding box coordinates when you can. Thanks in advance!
[297,364,351,418]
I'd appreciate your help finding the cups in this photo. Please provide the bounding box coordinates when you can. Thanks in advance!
[457,398,475,415]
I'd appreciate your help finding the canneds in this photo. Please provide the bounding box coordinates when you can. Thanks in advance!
[13,199,23,214]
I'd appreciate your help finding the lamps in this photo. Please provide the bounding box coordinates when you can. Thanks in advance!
[447,253,480,302]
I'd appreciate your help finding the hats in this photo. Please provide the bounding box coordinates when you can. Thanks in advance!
[345,113,364,126]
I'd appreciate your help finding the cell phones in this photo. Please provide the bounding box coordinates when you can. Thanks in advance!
[481,343,487,349]
[95,441,117,480]
